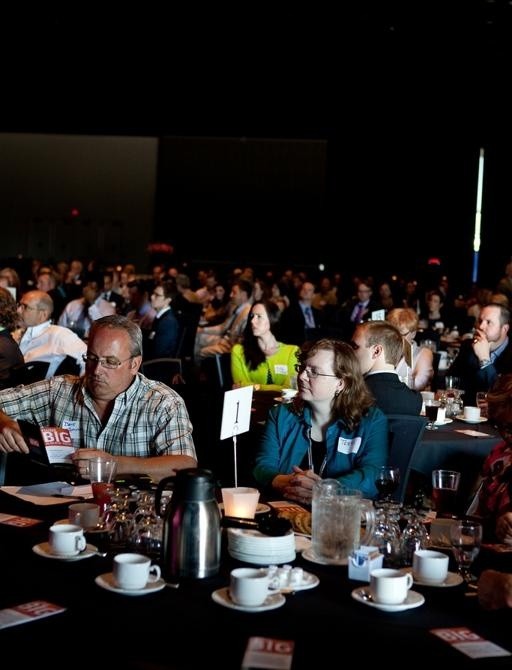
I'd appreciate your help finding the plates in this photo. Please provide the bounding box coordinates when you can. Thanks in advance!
[32,540,100,564]
[95,570,165,599]
[257,566,320,592]
[211,586,287,613]
[218,501,272,514]
[351,584,426,613]
[304,549,349,566]
[274,397,285,403]
[403,568,465,588]
[225,520,297,565]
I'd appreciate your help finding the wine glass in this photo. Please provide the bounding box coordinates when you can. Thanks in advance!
[101,488,163,552]
[415,373,491,431]
[376,468,490,583]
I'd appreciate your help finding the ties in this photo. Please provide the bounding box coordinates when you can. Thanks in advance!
[75,304,89,332]
[353,304,363,323]
[305,307,313,325]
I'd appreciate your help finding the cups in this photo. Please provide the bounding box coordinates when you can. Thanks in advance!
[48,502,100,557]
[311,477,377,560]
[89,456,116,498]
[227,568,283,607]
[222,486,262,521]
[270,565,304,586]
[111,553,161,589]
[420,339,448,371]
[282,387,298,400]
[369,569,413,604]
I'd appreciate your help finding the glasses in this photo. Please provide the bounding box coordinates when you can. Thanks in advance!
[152,292,166,298]
[16,302,46,312]
[400,331,410,338]
[294,365,339,379]
[82,352,141,368]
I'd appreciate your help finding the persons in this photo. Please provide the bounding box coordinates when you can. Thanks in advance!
[0,256,512,615]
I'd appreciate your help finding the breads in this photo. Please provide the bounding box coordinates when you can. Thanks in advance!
[274,505,366,541]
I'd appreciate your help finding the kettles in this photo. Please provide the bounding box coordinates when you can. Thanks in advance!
[153,468,223,583]
